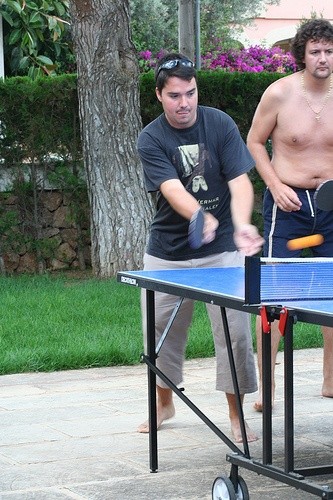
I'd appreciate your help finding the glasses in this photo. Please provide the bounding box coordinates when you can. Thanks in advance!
[155,59,193,84]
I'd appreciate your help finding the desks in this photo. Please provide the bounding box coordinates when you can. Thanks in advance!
[117,254,333,500]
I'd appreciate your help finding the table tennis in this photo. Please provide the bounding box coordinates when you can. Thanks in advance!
[288,234,323,251]
[314,180,333,211]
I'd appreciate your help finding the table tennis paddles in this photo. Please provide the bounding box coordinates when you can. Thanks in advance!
[189,209,204,249]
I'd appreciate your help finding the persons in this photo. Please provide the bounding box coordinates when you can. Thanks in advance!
[138,53,266,442]
[245,18,333,413]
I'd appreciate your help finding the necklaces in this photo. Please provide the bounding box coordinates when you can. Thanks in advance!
[299,72,333,127]
